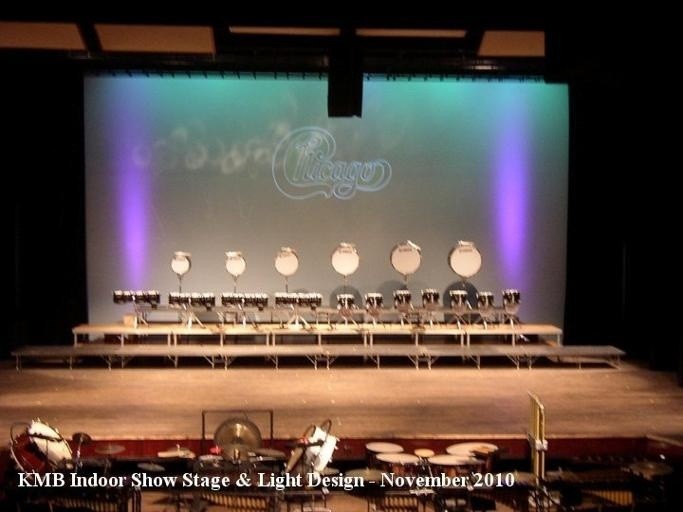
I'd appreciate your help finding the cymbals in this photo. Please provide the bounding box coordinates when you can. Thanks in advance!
[71,431,91,446]
[94,444,125,454]
[138,462,165,471]
[214,419,262,465]
[343,467,390,485]
[320,468,340,476]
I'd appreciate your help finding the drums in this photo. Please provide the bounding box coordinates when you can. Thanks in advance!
[283,424,337,485]
[331,240,481,278]
[363,439,498,511]
[337,289,522,321]
[112,288,322,312]
[8,421,74,484]
[199,454,225,471]
[169,248,300,277]
